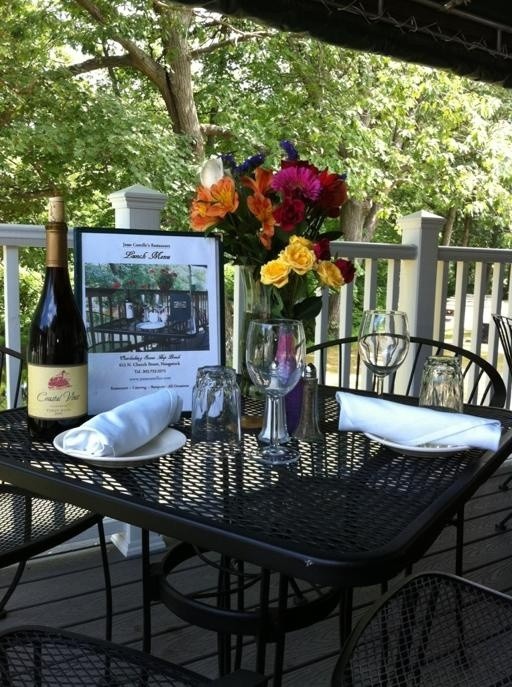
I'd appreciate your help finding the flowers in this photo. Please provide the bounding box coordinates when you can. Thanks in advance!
[184,137,359,297]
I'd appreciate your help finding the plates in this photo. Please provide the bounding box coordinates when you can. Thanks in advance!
[136,321,166,329]
[51,426,188,468]
[363,430,471,458]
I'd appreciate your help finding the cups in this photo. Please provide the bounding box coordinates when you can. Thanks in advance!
[419,354,464,414]
[191,366,242,460]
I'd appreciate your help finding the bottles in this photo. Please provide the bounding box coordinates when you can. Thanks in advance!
[291,363,325,442]
[26,195,89,443]
[124,288,135,324]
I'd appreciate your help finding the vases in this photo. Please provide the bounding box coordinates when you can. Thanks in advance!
[236,262,281,404]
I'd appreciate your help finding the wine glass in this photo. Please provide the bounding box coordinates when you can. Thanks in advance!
[244,319,308,466]
[358,309,411,401]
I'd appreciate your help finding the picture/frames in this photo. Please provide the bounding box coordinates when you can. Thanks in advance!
[70,225,229,420]
